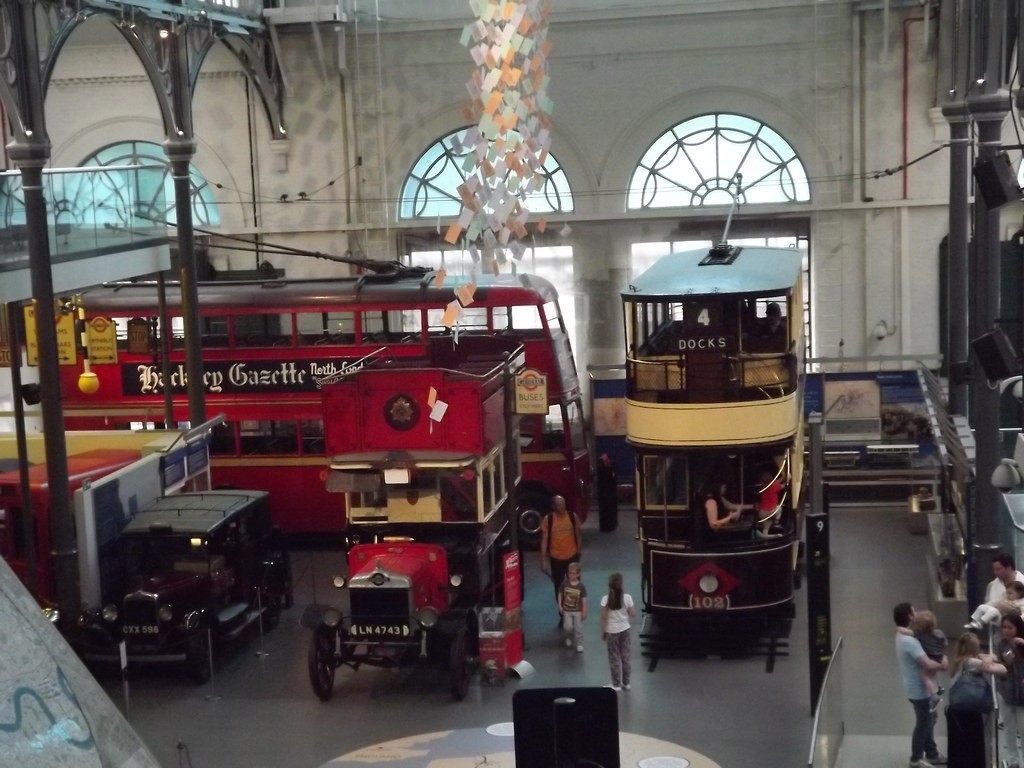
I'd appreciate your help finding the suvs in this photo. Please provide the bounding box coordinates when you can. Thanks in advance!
[76,488,294,683]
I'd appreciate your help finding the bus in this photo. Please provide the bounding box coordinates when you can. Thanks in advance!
[619,175,807,620]
[58,211,594,552]
[298,327,525,701]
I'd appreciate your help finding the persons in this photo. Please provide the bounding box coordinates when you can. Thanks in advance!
[599,573,636,691]
[893,603,947,768]
[757,473,788,535]
[699,475,756,543]
[944,634,1008,768]
[997,615,1024,707]
[962,552,1024,630]
[756,301,787,352]
[556,563,587,652]
[539,494,583,629]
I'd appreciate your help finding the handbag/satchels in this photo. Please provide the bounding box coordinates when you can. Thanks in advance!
[945,658,994,714]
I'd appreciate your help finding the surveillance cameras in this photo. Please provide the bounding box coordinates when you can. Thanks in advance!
[873,325,886,341]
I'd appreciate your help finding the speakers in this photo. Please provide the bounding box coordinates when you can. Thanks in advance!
[972,151,1024,210]
[970,328,1022,382]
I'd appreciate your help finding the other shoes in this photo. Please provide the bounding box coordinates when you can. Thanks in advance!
[603,684,621,692]
[938,687,945,695]
[560,618,563,627]
[925,752,948,764]
[577,646,583,652]
[621,683,631,690]
[964,624,973,629]
[910,760,935,768]
[566,638,572,647]
[971,621,983,629]
[928,697,942,713]
